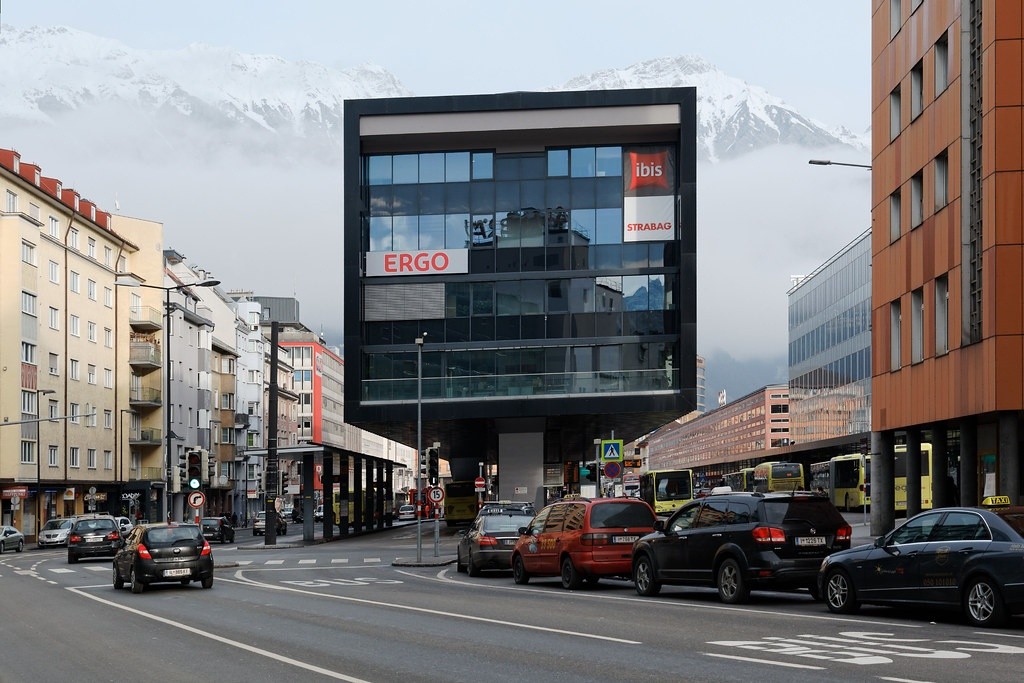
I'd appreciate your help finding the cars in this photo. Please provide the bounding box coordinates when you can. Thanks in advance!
[398,505,417,521]
[816,495,1024,628]
[316,504,323,522]
[67,516,128,564]
[37,517,77,549]
[0,526,25,554]
[198,516,236,544]
[113,516,133,538]
[252,510,288,536]
[110,520,215,595]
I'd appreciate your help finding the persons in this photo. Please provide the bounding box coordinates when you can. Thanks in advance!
[292,508,298,524]
[231,512,238,529]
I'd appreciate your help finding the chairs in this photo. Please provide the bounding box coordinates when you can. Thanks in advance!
[79,523,88,530]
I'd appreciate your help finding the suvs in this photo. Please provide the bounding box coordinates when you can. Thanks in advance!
[509,497,661,591]
[631,490,854,605]
[456,500,539,577]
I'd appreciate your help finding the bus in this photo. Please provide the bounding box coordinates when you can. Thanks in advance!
[638,468,696,517]
[333,488,387,528]
[443,479,486,526]
[695,439,932,512]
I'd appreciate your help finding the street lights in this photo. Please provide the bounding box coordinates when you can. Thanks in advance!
[208,418,222,517]
[113,278,222,521]
[243,428,258,529]
[35,388,56,537]
[277,435,289,469]
[415,336,425,564]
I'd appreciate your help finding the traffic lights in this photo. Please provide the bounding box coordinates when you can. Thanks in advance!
[187,450,203,490]
[179,452,188,482]
[256,471,265,491]
[278,470,289,496]
[201,448,216,483]
[420,446,431,478]
[427,448,438,486]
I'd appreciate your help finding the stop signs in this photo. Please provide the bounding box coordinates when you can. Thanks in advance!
[475,477,485,488]
[428,487,445,503]
